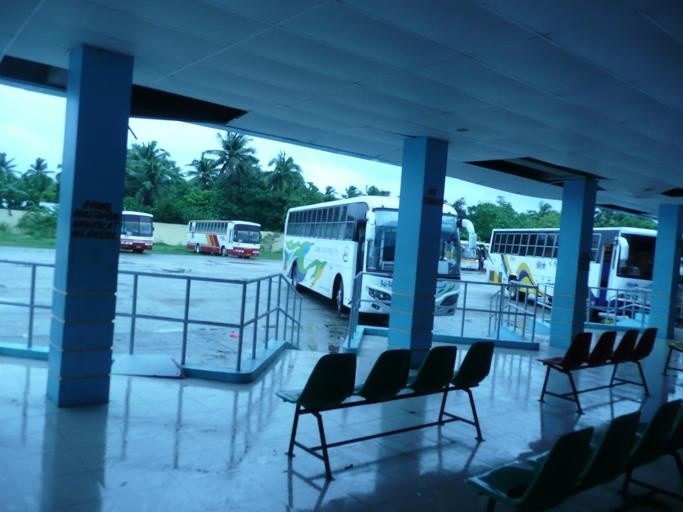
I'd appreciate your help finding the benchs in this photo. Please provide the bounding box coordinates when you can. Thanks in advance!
[463,398,682,511]
[276,339,496,480]
[535,328,658,416]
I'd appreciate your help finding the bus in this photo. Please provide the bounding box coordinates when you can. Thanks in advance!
[486,226,657,322]
[186,220,261,259]
[460,240,490,270]
[119,210,154,253]
[282,195,477,319]
